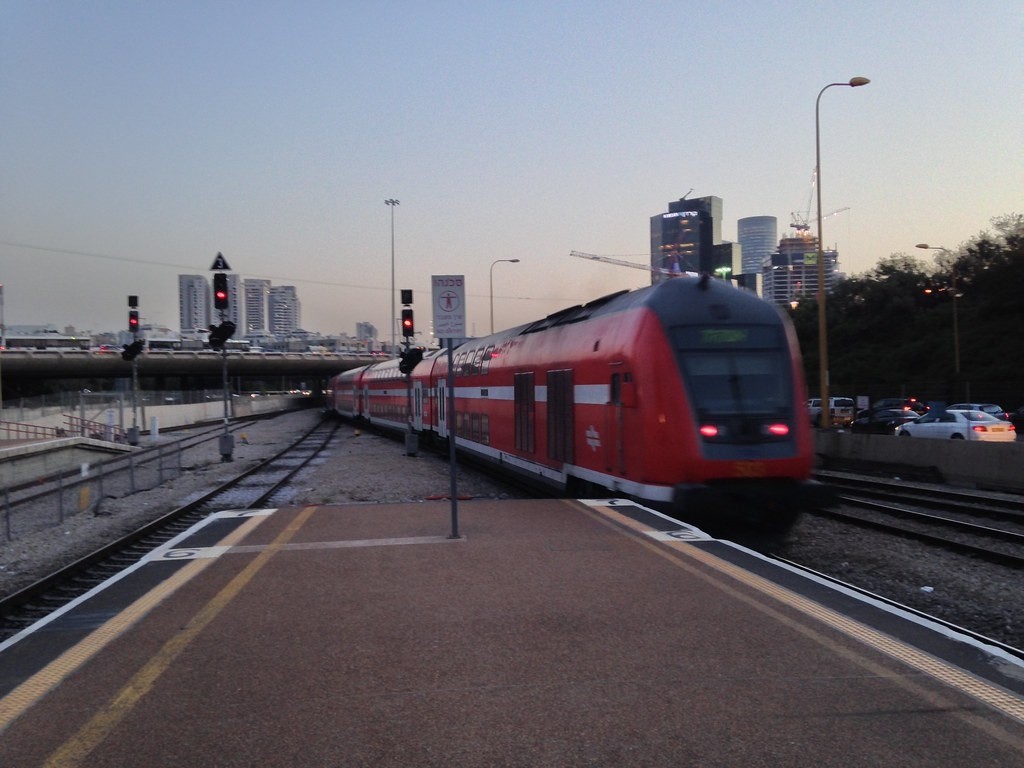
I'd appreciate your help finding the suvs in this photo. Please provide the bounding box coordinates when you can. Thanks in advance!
[946,403,1024,422]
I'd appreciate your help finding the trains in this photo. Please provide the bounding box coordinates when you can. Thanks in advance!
[325,274,814,522]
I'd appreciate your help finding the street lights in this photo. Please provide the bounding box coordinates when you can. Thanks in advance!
[816,77,870,430]
[490,260,520,333]
[384,199,402,360]
[916,243,963,403]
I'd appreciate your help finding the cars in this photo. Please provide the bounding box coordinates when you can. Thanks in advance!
[850,398,931,435]
[894,409,1017,442]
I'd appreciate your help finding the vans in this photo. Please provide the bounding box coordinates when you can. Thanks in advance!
[807,397,855,425]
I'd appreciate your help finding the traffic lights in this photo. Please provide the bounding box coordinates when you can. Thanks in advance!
[402,290,412,303]
[402,310,413,336]
[129,296,138,307]
[214,274,228,310]
[128,310,139,333]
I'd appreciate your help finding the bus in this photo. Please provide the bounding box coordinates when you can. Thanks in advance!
[1,333,91,353]
[146,337,250,354]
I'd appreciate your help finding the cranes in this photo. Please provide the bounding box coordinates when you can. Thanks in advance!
[571,251,691,277]
[789,167,849,238]
